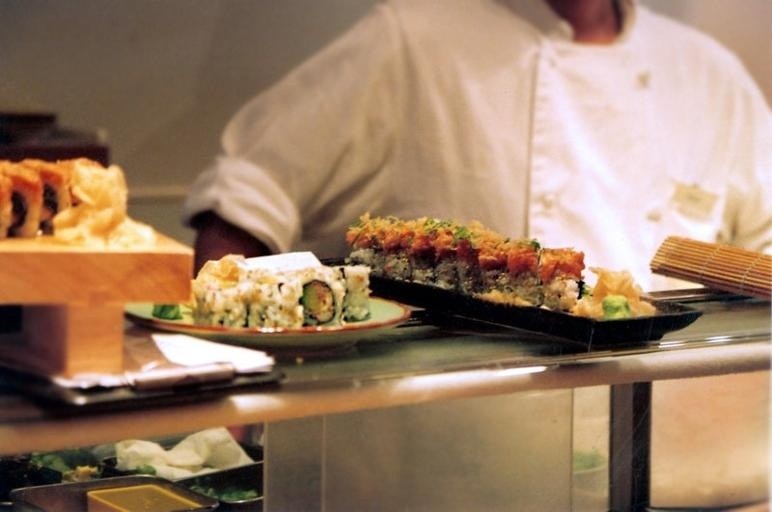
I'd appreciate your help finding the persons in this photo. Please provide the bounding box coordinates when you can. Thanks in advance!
[182,0,772,293]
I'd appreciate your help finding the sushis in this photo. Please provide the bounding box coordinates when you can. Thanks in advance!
[187,251,373,330]
[343,211,657,321]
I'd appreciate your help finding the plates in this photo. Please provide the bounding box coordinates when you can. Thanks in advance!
[319,255,704,344]
[124,294,409,347]
